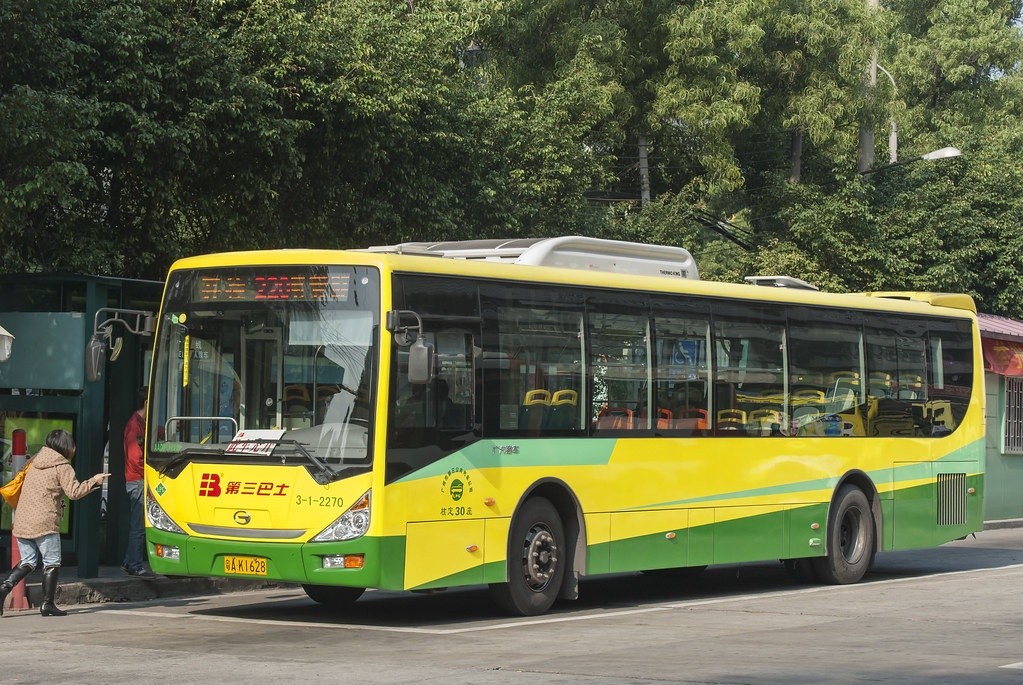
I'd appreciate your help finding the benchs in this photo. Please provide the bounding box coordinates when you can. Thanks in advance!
[278,382,369,411]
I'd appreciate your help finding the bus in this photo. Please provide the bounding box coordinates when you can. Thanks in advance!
[85,235,987,615]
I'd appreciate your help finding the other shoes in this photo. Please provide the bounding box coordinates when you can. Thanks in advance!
[121,562,129,573]
[128,568,156,579]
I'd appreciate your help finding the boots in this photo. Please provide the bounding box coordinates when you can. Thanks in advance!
[40,567,67,616]
[0,562,32,616]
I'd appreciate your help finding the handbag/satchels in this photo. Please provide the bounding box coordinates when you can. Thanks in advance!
[0,454,37,510]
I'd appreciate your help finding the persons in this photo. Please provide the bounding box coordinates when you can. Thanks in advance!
[0,430,112,618]
[120,386,148,576]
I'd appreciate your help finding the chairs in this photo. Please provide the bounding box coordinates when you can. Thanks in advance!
[516,387,578,431]
[676,409,708,430]
[598,408,633,428]
[719,372,927,437]
[641,409,674,432]
